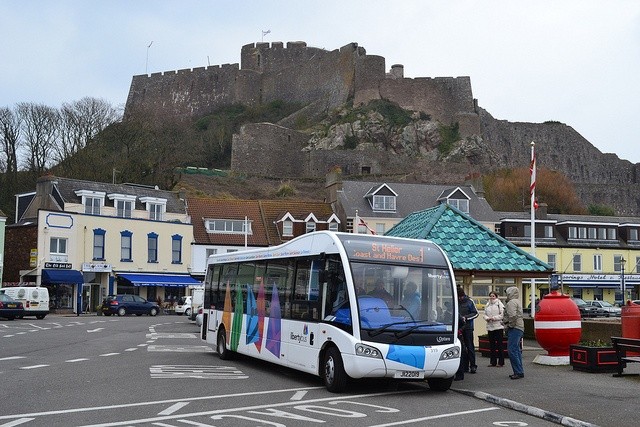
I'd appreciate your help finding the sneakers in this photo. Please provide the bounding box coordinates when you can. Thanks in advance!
[511,374,524,378]
[471,366,477,372]
[496,363,503,366]
[454,376,464,380]
[509,373,516,377]
[488,363,497,366]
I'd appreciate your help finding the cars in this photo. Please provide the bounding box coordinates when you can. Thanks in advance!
[101,294,160,317]
[174,296,193,317]
[585,300,623,318]
[195,305,203,326]
[0,292,24,322]
[572,298,601,318]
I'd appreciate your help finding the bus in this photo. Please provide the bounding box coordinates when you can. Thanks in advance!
[200,230,463,394]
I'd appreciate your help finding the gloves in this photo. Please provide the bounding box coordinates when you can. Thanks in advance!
[461,316,467,323]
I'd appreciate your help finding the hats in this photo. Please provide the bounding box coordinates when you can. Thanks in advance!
[458,287,464,295]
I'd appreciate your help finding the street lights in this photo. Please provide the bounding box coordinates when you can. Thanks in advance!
[620,257,627,307]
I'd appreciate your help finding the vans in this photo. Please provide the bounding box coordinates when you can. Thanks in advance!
[187,285,205,322]
[0,287,51,320]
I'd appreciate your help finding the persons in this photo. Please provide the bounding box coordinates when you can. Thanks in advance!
[366,278,394,315]
[441,298,453,325]
[456,304,465,381]
[502,285,526,380]
[483,291,505,369]
[462,285,479,374]
[401,282,421,322]
